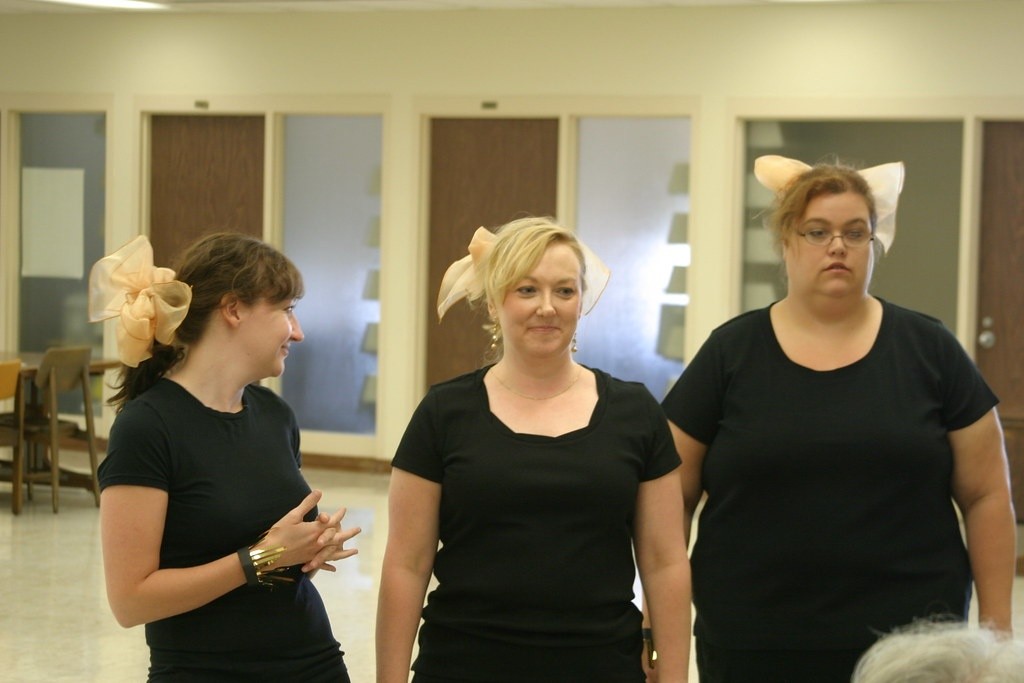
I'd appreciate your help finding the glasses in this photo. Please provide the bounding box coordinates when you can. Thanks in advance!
[801,228,875,248]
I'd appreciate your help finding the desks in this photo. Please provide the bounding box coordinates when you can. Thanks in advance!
[0,350,123,501]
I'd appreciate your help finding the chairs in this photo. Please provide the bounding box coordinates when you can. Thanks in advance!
[0,357,25,516]
[0,346,102,513]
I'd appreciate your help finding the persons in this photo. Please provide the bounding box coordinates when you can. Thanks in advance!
[854,611,1024,683]
[640,164,1017,683]
[373,213,692,683]
[93,234,361,683]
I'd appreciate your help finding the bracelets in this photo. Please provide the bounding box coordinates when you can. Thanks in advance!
[237,530,295,589]
[642,628,656,668]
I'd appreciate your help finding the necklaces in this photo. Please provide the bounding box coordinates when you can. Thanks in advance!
[490,366,584,399]
